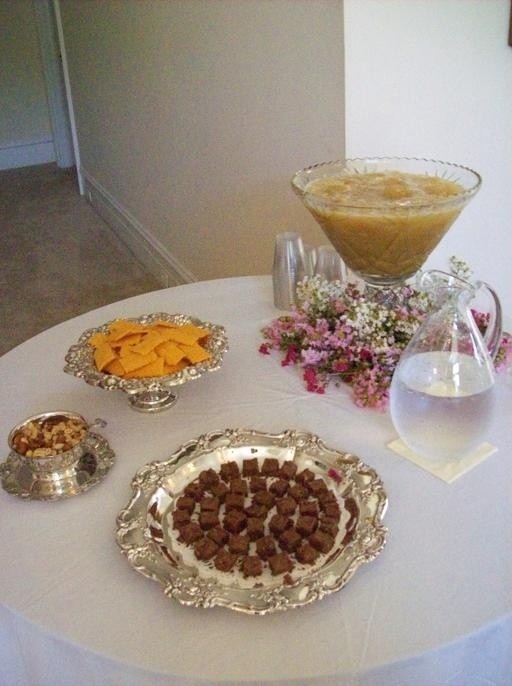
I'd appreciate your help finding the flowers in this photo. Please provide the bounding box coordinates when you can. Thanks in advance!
[257,255,512,410]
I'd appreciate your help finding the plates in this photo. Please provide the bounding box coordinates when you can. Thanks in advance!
[0,435,119,505]
[116,426,389,618]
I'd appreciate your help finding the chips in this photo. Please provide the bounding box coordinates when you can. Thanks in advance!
[88,319,211,377]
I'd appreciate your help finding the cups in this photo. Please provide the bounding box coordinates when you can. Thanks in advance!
[9,410,106,478]
[269,232,344,313]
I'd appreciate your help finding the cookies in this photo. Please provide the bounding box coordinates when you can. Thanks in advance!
[172,458,341,577]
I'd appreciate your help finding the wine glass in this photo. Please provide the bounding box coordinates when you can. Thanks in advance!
[289,156,481,313]
[64,314,229,414]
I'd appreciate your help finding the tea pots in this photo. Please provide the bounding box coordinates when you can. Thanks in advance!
[387,265,502,465]
